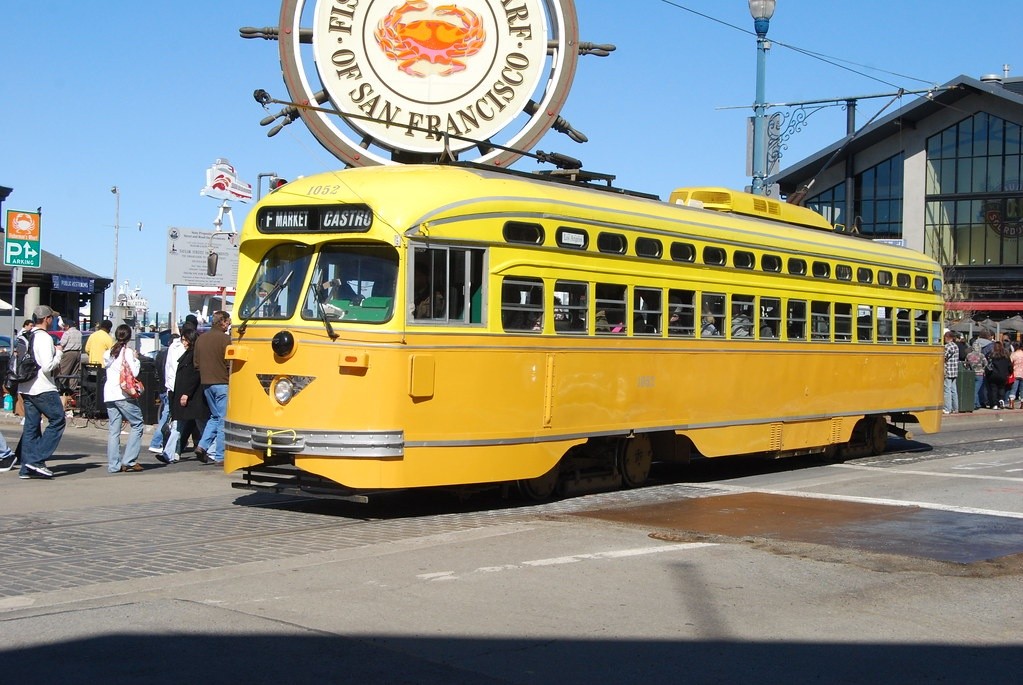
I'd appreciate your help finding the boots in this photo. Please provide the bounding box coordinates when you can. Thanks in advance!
[1008,397,1014,409]
[1020,399,1023,409]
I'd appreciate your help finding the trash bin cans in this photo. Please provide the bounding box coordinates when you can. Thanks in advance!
[957,361,975,412]
[136,361,164,424]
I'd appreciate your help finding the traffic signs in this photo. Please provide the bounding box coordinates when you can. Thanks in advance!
[6,239,40,264]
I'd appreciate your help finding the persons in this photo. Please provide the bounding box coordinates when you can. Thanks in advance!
[0,303,233,479]
[942,328,1023,414]
[371,266,927,344]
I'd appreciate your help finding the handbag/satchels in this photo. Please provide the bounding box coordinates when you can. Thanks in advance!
[119,348,146,398]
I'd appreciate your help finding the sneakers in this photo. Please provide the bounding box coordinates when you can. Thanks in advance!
[0,455,17,472]
[25,462,53,476]
[19,472,51,479]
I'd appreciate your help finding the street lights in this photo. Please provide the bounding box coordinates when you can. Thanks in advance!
[110,185,121,304]
[746,0,779,197]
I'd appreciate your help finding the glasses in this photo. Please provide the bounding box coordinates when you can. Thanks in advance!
[180,339,187,343]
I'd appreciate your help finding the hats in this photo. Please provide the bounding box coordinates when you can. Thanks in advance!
[63,319,75,327]
[33,304,59,319]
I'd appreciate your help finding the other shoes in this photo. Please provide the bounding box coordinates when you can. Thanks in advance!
[121,462,144,471]
[155,452,179,464]
[993,404,998,410]
[943,408,950,414]
[149,446,164,453]
[13,461,22,469]
[998,400,1006,409]
[194,446,208,463]
[951,408,958,414]
[986,404,990,409]
[215,459,225,466]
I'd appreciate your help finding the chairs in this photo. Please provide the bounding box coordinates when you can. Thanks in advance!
[531,227,925,342]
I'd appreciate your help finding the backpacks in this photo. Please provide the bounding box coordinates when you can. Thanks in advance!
[8,328,50,383]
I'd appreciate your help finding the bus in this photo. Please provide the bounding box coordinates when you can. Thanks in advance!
[221,157,947,506]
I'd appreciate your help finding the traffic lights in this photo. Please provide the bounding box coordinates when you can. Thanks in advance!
[268,177,288,193]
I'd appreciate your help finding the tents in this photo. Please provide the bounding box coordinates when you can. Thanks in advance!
[948,314,1023,342]
